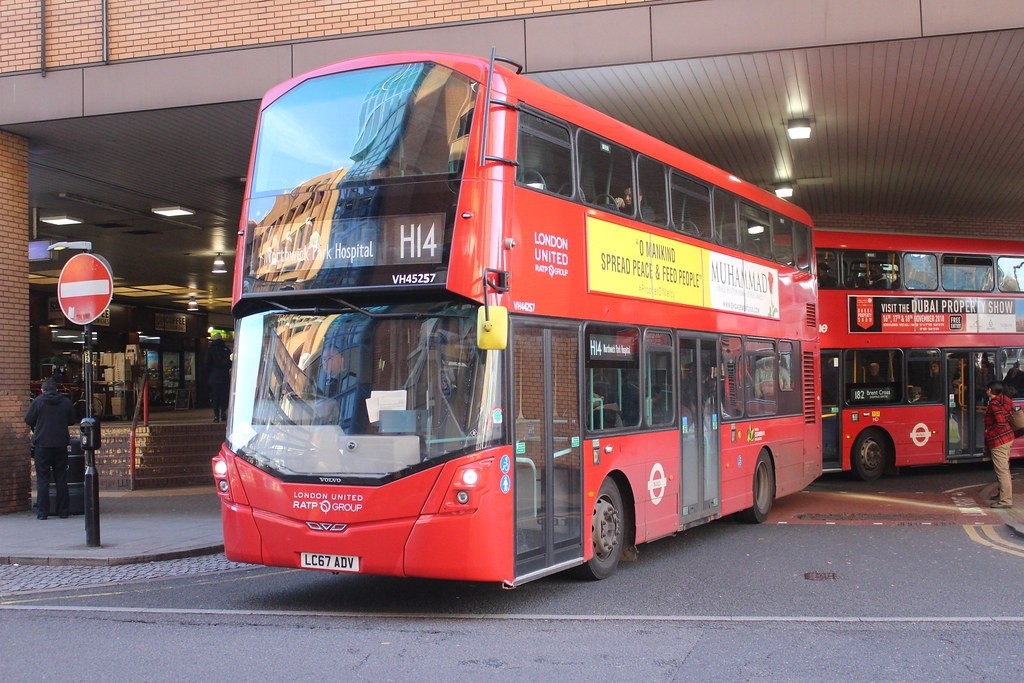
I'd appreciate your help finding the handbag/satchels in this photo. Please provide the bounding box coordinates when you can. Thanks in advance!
[1005,410,1024,431]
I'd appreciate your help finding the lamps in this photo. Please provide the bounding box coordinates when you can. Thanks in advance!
[39,216,84,225]
[186,296,199,311]
[747,220,764,234]
[151,206,195,217]
[212,253,228,273]
[775,181,793,197]
[787,118,811,140]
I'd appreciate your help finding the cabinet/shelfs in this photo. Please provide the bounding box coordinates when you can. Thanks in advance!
[150,378,179,406]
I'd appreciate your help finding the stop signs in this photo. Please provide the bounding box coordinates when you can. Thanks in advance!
[57,253,114,324]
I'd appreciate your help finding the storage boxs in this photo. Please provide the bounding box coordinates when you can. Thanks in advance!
[101,397,126,415]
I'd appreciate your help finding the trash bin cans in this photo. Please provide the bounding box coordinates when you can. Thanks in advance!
[48,438,85,516]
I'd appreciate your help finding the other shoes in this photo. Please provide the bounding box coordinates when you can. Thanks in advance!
[990,502,1011,508]
[36,514,47,520]
[58,512,68,518]
[990,495,1001,501]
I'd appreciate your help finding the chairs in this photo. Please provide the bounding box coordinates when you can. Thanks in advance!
[518,168,763,256]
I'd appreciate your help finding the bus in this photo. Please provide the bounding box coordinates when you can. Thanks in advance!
[723,227,1024,481]
[212,46,823,588]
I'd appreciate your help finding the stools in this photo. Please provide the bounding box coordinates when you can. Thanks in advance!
[100,344,141,390]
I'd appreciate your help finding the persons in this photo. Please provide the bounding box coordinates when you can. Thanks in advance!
[818,257,1024,292]
[587,360,731,432]
[25,380,77,520]
[614,184,655,222]
[984,381,1015,508]
[185,359,191,375]
[747,219,768,256]
[166,360,176,379]
[823,353,1024,459]
[312,344,360,435]
[46,368,65,383]
[205,334,232,422]
